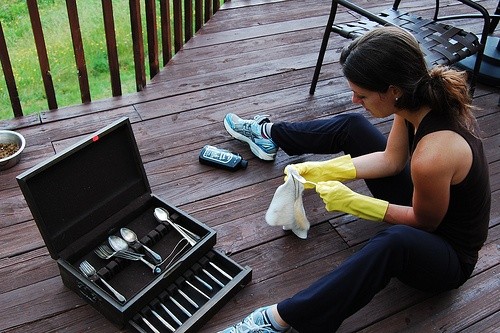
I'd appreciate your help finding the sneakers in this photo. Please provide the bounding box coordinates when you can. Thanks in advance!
[224,113,279,160]
[217,306,293,333]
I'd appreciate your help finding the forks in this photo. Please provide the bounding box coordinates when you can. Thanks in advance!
[93,241,145,265]
[79,261,125,303]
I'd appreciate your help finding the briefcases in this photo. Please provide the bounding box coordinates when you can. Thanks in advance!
[16,116,253,333]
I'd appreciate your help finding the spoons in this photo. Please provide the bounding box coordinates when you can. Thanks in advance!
[155,208,201,247]
[291,172,317,187]
[109,228,162,274]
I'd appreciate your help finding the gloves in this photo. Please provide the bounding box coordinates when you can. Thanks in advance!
[284,154,356,190]
[316,181,389,223]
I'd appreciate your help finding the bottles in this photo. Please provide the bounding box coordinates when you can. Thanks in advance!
[198,144,248,172]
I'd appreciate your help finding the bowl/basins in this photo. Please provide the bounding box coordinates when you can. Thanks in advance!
[0,129,26,171]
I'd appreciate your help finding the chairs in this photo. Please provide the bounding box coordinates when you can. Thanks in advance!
[309,0,491,106]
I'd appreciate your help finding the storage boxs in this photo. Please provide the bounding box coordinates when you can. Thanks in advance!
[15,116,253,333]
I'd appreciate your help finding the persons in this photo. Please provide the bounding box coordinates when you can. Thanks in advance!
[217,27,492,333]
